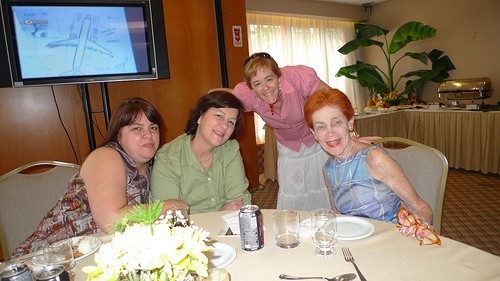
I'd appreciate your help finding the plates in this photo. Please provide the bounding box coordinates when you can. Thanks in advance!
[321,216,376,240]
[203,243,236,269]
[32,235,102,265]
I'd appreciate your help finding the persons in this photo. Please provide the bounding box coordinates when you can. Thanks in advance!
[304,87,433,236]
[150,91,251,215]
[231,52,332,210]
[9,97,190,260]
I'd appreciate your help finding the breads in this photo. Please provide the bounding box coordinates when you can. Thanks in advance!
[78,237,93,253]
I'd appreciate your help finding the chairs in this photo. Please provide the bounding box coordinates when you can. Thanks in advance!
[375,137,449,235]
[0,160,81,262]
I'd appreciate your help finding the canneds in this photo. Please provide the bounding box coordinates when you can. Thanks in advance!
[239,205,264,252]
[0,263,71,281]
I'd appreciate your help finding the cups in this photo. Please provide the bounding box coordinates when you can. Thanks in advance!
[272,209,300,249]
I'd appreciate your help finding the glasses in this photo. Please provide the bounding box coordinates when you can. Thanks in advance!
[396,206,441,246]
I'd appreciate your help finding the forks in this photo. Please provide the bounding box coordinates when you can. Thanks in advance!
[342,246,367,281]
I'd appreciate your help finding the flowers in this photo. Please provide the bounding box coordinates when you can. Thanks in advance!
[84,200,213,281]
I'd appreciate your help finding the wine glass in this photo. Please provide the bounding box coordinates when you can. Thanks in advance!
[44,234,75,280]
[312,209,338,257]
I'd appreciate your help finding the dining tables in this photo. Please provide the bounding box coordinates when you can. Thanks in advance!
[0,208,500,281]
[264,107,500,182]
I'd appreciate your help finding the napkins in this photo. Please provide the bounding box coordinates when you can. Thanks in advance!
[221,209,266,235]
[285,216,328,236]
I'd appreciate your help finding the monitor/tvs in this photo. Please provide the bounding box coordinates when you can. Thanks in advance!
[0,0,172,88]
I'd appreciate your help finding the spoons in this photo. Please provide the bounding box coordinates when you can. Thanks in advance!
[280,274,356,281]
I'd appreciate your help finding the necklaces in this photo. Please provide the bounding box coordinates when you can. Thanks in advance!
[199,155,212,163]
[333,142,353,187]
